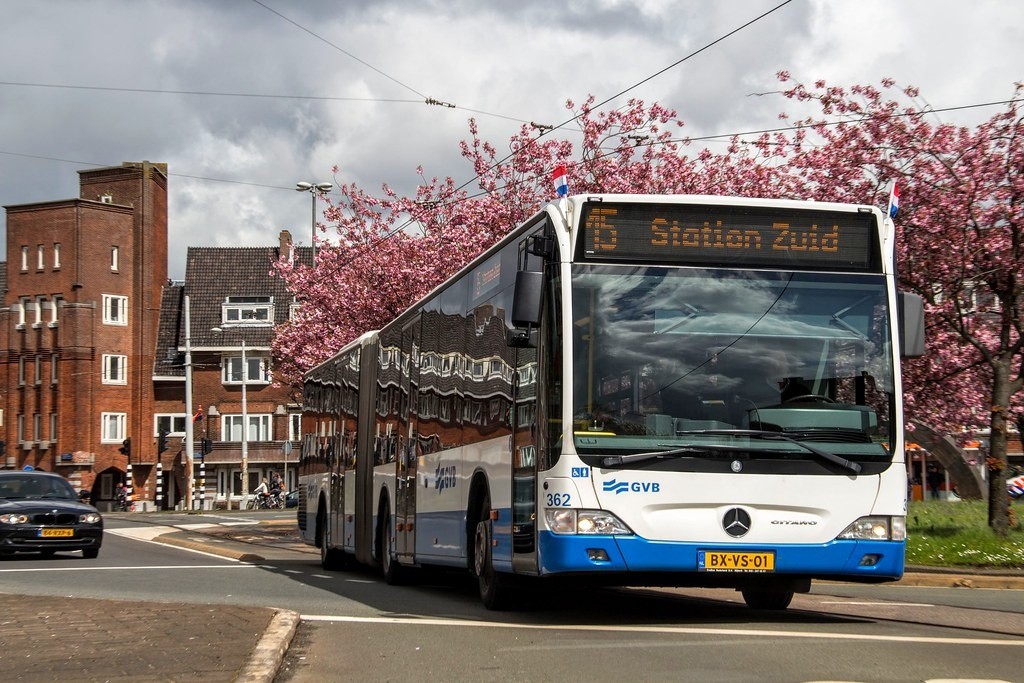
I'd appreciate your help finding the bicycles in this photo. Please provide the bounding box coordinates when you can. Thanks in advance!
[245,489,286,510]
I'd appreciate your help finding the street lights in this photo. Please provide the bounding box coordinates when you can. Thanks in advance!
[296,178,334,276]
[210,326,250,508]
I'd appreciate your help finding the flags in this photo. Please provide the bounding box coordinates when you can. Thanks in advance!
[552,166,568,196]
[891,184,898,217]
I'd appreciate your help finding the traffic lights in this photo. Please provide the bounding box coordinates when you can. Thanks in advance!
[160,429,171,452]
[119,440,129,455]
[206,439,212,454]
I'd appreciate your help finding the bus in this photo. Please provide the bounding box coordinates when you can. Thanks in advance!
[296,189,928,611]
[904,439,989,505]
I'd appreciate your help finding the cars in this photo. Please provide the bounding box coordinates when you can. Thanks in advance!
[0,468,104,559]
[284,490,298,509]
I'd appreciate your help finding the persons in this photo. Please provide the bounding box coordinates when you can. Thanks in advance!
[115,482,127,508]
[930,466,942,499]
[254,472,285,506]
[907,472,914,500]
